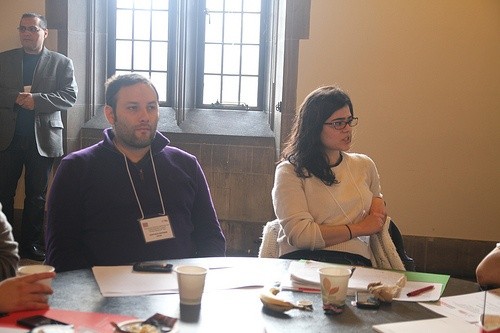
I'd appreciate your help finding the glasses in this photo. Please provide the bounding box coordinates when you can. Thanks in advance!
[17,26,43,33]
[321,116,358,130]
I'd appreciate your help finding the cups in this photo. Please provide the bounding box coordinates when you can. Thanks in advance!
[30,324,75,333]
[16,265,55,305]
[174,265,209,306]
[318,266,352,306]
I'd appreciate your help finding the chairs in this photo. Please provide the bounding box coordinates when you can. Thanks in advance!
[258,213,414,271]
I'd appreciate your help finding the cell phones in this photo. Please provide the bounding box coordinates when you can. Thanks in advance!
[355,289,380,308]
[133,262,173,271]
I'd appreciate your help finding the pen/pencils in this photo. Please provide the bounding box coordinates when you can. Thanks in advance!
[407,286,433,296]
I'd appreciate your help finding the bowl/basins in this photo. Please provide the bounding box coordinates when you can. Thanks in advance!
[115,319,179,333]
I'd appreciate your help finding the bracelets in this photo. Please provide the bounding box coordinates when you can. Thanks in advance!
[344,223,354,240]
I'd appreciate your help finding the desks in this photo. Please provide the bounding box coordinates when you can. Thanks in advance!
[49,256,500,333]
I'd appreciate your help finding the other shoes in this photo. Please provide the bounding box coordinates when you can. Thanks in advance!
[21,246,46,262]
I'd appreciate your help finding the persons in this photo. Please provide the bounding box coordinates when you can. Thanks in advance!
[0,200,57,312]
[271,83,388,268]
[43,72,227,272]
[476,240,500,290]
[0,11,78,263]
